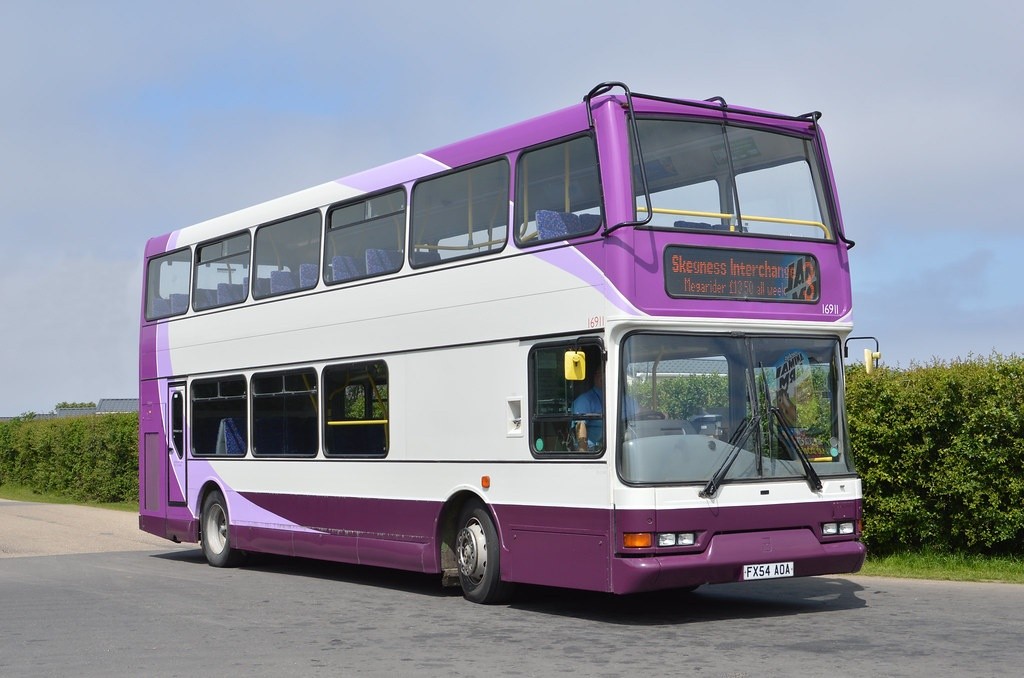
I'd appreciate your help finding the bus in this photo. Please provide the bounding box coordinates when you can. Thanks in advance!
[136,81,882,603]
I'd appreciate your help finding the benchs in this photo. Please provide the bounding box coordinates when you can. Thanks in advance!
[188,417,384,456]
[534,209,603,241]
[153,250,440,317]
[676,221,748,233]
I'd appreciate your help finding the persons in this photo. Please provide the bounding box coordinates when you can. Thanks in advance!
[569,363,645,452]
